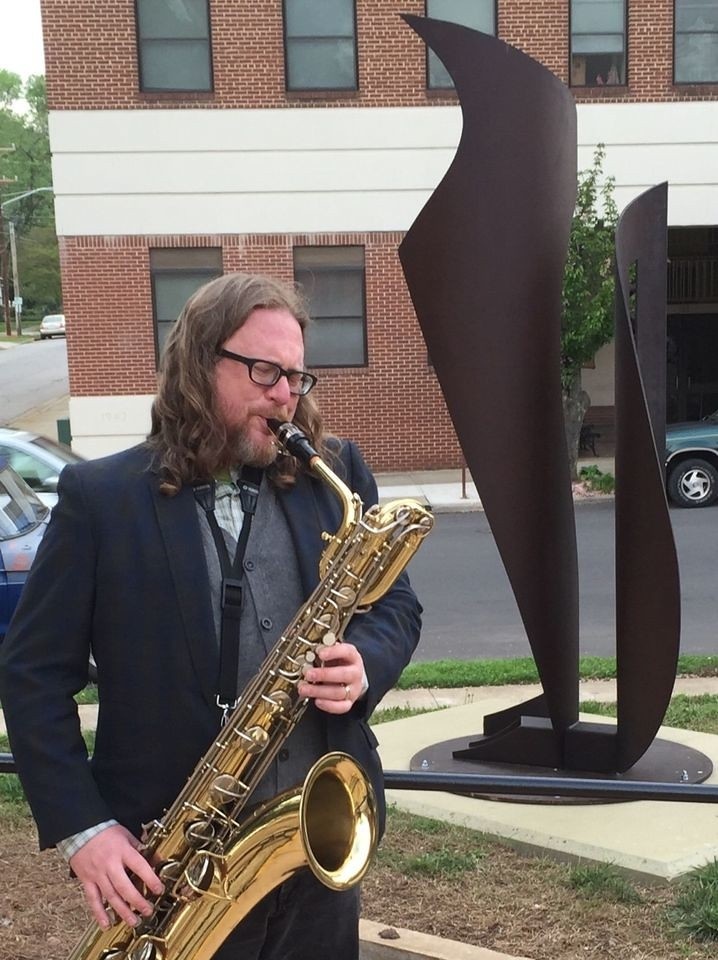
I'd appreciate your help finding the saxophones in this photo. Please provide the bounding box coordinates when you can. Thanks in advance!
[68,419,434,960]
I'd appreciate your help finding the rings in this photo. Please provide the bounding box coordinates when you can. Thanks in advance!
[345,686,350,700]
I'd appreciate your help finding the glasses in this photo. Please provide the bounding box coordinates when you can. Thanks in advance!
[217,348,318,396]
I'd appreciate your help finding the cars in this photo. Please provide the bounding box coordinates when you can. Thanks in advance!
[39,314,66,339]
[665,409,718,509]
[0,426,87,645]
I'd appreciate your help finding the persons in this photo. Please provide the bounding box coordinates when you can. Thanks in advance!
[0,274,424,960]
